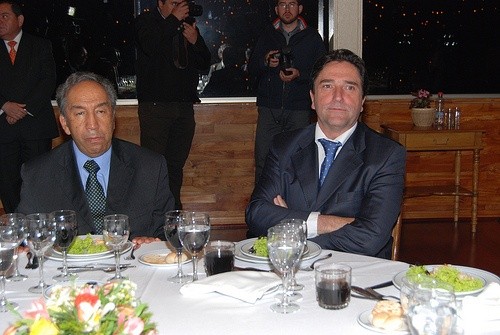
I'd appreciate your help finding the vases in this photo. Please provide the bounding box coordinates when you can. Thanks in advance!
[411,107,437,126]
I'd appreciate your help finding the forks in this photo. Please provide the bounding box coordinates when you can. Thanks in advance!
[298,253,332,272]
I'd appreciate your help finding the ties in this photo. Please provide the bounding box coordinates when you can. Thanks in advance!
[83,159,106,235]
[318,138,342,188]
[7,40,18,65]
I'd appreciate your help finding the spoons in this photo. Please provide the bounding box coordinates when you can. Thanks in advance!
[60,266,127,273]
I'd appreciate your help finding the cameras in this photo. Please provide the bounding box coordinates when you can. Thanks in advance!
[179,0,203,25]
[272,47,293,76]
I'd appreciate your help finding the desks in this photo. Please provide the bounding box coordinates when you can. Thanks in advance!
[379,121,486,233]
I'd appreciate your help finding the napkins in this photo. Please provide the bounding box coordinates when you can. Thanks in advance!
[179,270,312,304]
[457,283,500,335]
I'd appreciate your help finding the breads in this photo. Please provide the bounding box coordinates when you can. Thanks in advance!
[370,299,410,332]
[142,247,203,263]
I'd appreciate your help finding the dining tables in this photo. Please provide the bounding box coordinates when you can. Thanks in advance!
[0,241,500,335]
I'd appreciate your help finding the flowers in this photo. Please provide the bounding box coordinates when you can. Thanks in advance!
[4,278,157,335]
[408,89,435,110]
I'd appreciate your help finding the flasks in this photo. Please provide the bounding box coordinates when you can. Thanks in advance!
[177,212,211,284]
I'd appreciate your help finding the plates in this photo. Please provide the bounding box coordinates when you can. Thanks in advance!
[43,280,103,299]
[240,240,309,259]
[401,271,487,296]
[356,308,412,335]
[232,237,323,264]
[44,239,134,262]
[52,234,115,259]
[138,248,193,267]
[392,264,500,300]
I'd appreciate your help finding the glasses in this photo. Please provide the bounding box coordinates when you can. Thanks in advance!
[277,3,297,9]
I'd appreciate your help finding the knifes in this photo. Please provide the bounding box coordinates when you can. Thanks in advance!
[368,281,394,290]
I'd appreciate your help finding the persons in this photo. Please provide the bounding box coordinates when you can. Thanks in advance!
[125,0,211,186]
[244,49,407,260]
[11,71,180,244]
[200,41,253,95]
[246,0,328,191]
[0,0,59,213]
[53,44,87,87]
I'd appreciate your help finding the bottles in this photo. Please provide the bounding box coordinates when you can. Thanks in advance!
[434,92,445,123]
[446,107,459,119]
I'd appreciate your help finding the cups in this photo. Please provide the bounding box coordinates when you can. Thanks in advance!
[314,262,352,310]
[204,240,235,277]
[405,279,458,335]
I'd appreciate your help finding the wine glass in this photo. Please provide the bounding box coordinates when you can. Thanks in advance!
[103,213,130,282]
[163,210,195,283]
[0,212,29,282]
[267,218,307,314]
[49,209,79,282]
[22,213,57,294]
[0,225,20,313]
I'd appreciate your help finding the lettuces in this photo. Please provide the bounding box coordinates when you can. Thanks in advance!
[254,237,271,257]
[405,264,483,292]
[67,233,109,254]
